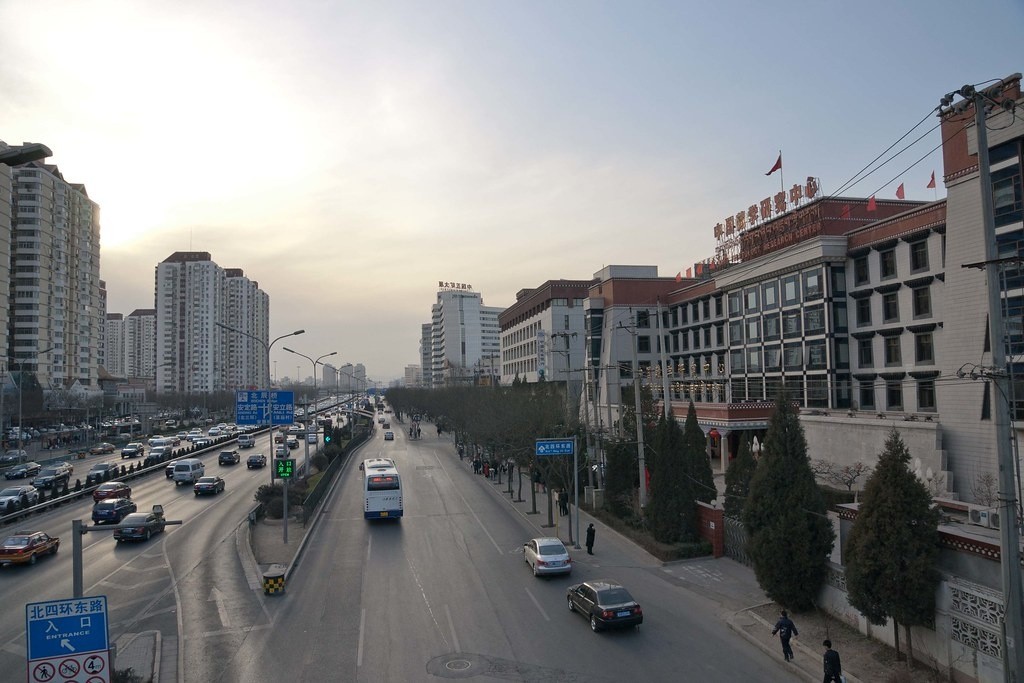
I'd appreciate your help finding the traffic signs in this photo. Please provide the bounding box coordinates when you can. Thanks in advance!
[25,594,112,683]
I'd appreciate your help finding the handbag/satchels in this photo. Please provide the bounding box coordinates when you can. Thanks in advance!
[839,674,846,683]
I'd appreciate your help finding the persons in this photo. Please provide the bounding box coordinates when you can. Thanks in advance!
[436,423,441,436]
[534,472,547,493]
[771,611,799,662]
[48,436,79,448]
[417,428,421,437]
[4,442,9,452]
[822,640,842,683]
[586,523,595,555]
[555,488,568,517]
[409,427,413,436]
[473,447,507,478]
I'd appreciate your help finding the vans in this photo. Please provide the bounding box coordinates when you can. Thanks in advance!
[172,457,206,487]
[237,434,255,449]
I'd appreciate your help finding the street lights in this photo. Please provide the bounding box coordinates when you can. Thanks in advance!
[0,346,55,464]
[215,321,306,492]
[316,361,367,431]
[282,347,337,452]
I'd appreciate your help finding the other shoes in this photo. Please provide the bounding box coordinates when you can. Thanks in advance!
[784,658,789,662]
[589,553,594,555]
[790,655,793,659]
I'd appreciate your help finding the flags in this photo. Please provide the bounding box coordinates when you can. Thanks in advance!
[696,262,702,274]
[685,268,691,278]
[842,204,850,218]
[867,196,876,211]
[928,173,935,188]
[897,184,904,199]
[676,272,681,282]
[765,156,781,175]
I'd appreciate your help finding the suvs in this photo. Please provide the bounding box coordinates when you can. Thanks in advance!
[120,442,145,460]
[218,450,241,465]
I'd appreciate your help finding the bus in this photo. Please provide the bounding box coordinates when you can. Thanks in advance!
[358,458,403,522]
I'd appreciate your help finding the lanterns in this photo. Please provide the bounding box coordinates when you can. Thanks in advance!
[710,431,719,445]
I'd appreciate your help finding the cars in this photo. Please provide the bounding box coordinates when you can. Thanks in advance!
[412,414,421,421]
[4,461,42,480]
[113,512,166,544]
[0,394,370,513]
[0,484,40,516]
[384,431,394,440]
[89,442,116,455]
[0,530,61,568]
[86,460,120,484]
[383,421,390,429]
[193,476,226,496]
[378,417,385,423]
[565,578,644,633]
[0,449,28,466]
[92,481,132,503]
[523,536,572,578]
[246,454,267,470]
[91,497,138,525]
[29,465,71,490]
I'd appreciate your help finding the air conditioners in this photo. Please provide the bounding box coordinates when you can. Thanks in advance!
[967,506,989,528]
[988,507,1000,529]
